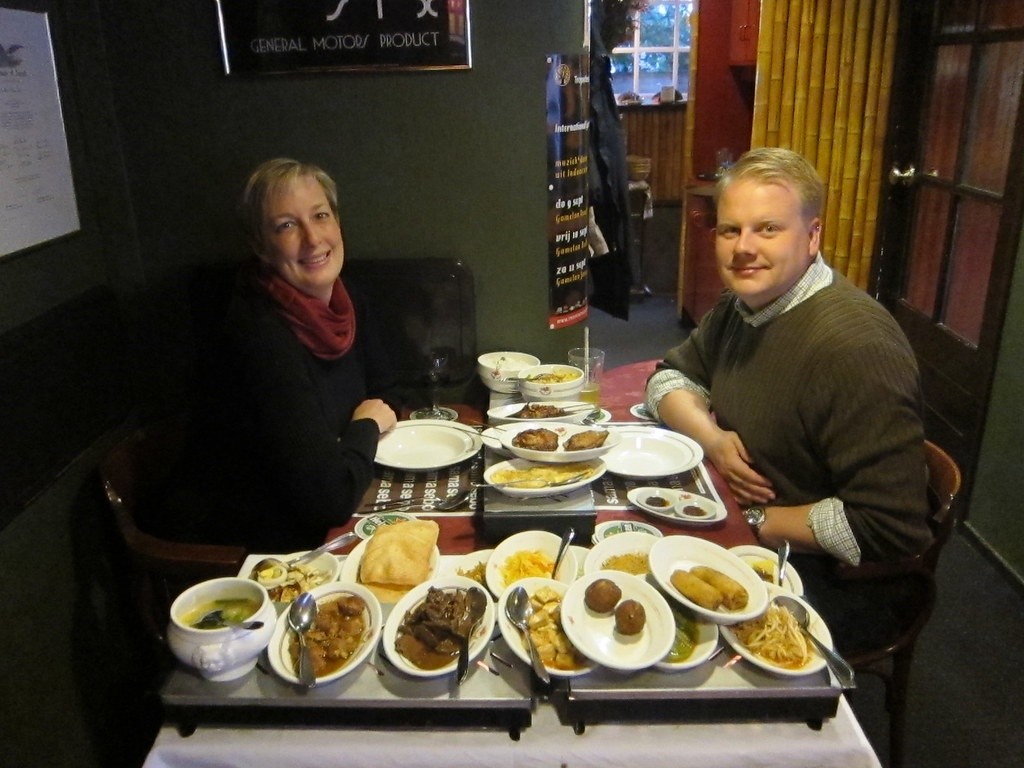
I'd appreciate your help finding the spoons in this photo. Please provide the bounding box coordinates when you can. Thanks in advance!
[456,587,487,686]
[505,587,551,685]
[287,592,318,689]
[770,596,854,685]
[364,490,471,510]
[251,531,358,588]
[194,609,265,630]
[470,472,589,487]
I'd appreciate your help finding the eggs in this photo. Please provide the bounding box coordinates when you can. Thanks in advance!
[257,566,288,586]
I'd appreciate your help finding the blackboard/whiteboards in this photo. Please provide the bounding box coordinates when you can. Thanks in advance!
[0,7,81,267]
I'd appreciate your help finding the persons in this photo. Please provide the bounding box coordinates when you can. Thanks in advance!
[140,157,403,635]
[643,148,931,661]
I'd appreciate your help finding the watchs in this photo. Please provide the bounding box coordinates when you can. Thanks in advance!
[743,505,768,542]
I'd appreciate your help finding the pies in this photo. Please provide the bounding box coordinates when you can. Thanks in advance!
[511,428,608,454]
[490,463,595,489]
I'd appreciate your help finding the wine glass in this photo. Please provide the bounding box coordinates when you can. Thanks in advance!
[414,348,453,420]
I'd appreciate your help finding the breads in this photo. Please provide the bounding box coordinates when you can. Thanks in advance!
[360,520,440,586]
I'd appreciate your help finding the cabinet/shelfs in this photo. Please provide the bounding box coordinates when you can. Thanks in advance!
[685,176,724,327]
[727,0,760,67]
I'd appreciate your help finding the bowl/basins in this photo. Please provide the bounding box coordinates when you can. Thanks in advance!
[166,577,278,683]
[374,419,483,472]
[600,426,704,480]
[651,622,718,672]
[518,364,585,403]
[477,351,541,394]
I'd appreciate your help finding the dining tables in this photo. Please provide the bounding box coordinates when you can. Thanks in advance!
[131,357,882,768]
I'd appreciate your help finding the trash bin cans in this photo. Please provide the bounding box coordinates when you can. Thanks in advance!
[626,179,653,300]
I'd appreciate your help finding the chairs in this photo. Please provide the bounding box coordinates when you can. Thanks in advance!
[94,412,244,727]
[843,443,959,768]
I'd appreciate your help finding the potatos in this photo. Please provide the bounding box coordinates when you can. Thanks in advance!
[584,578,645,635]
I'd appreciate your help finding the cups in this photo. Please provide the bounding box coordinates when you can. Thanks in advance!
[714,149,734,176]
[567,347,606,404]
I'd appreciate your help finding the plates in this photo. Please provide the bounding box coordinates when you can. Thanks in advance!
[649,534,768,626]
[340,534,440,628]
[384,575,496,677]
[569,545,590,582]
[497,577,598,678]
[483,457,608,499]
[626,487,728,527]
[485,530,578,600]
[560,570,675,672]
[727,544,804,597]
[486,401,597,421]
[719,581,834,678]
[481,422,622,464]
[277,550,340,588]
[266,580,382,687]
[584,531,659,587]
